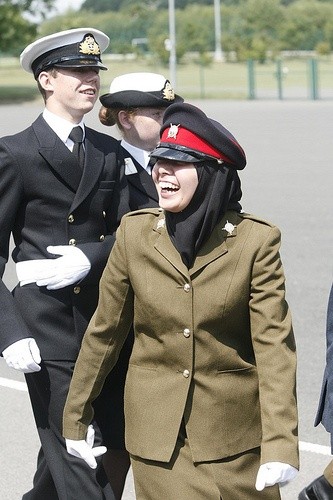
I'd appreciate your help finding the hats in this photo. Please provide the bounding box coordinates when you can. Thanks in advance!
[148,102,247,170]
[19,28,110,79]
[98,71,183,110]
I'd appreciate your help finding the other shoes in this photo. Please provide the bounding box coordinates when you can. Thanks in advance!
[299,475,333,500]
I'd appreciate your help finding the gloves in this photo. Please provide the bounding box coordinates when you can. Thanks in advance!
[65,424,106,470]
[1,337,42,374]
[32,245,90,291]
[255,462,298,491]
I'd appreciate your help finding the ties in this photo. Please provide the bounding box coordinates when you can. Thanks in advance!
[69,127,86,170]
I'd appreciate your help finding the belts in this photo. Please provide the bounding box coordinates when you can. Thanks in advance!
[16,260,56,286]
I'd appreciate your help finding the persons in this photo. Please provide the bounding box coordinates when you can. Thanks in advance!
[0,29,160,500]
[97,73,184,178]
[63,103,300,500]
[298,286,333,500]
